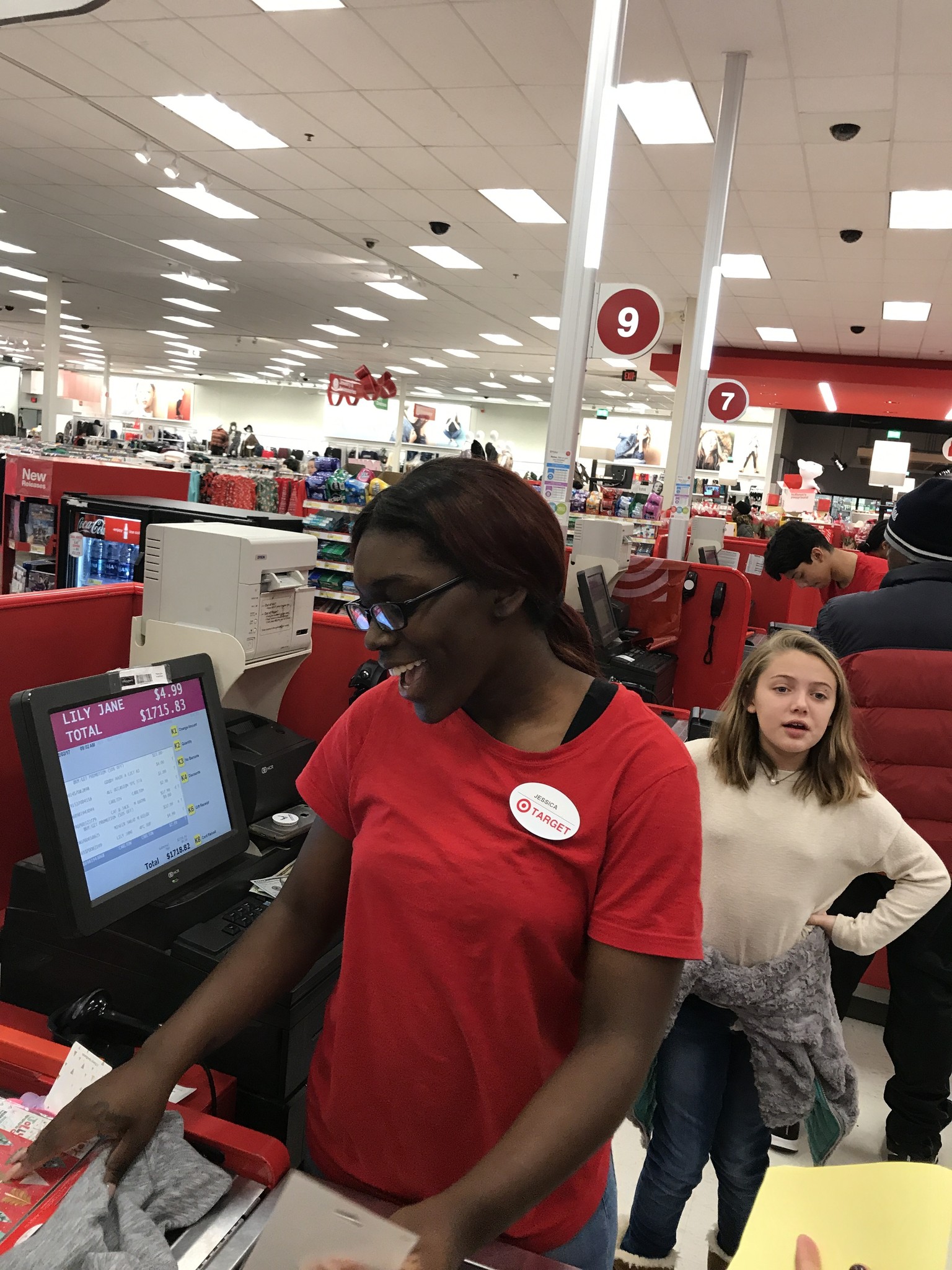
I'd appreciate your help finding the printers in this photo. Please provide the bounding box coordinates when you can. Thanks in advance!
[147,523,319,664]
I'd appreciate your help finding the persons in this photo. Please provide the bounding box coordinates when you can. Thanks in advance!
[390,405,462,448]
[695,429,759,474]
[209,422,260,457]
[732,501,754,538]
[764,519,890,604]
[652,481,663,496]
[130,382,185,420]
[307,458,317,475]
[768,478,951,1164]
[7,458,703,1270]
[614,422,651,460]
[611,629,951,1269]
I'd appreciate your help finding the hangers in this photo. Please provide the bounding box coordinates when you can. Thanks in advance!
[188,463,307,489]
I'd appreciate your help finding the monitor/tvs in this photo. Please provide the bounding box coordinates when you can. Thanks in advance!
[576,565,620,646]
[9,653,249,939]
[697,546,719,566]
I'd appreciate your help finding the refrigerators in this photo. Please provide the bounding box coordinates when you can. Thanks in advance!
[56,492,303,592]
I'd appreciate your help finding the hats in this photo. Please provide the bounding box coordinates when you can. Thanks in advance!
[883,477,952,565]
[733,501,751,515]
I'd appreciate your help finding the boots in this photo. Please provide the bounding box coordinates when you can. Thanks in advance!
[612,1242,679,1270]
[705,1227,733,1270]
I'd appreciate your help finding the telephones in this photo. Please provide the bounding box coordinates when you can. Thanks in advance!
[710,581,727,619]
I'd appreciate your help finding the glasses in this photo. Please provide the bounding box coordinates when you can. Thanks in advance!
[346,573,472,635]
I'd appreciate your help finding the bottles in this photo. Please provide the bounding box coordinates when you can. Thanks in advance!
[88,538,140,586]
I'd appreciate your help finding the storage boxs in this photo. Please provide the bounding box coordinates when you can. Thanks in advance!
[776,473,816,512]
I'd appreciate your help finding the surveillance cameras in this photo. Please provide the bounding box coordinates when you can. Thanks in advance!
[428,222,451,236]
[850,326,865,334]
[363,238,380,249]
[840,229,863,243]
[81,324,90,329]
[830,123,861,142]
[4,305,14,311]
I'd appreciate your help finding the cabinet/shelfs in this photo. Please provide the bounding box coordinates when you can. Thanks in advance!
[295,495,366,616]
[567,512,664,567]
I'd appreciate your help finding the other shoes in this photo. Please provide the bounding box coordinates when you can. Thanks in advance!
[889,1145,941,1166]
[771,1123,802,1156]
